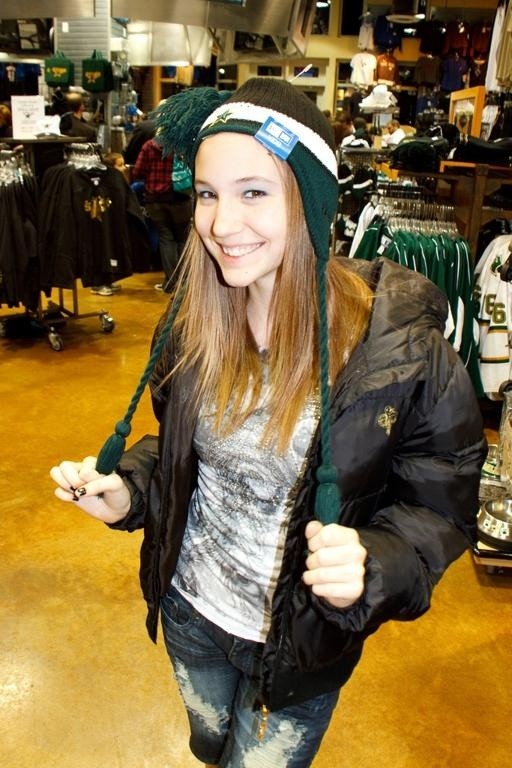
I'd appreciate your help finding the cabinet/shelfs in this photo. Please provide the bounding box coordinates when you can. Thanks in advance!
[337,59,419,122]
[401,164,511,251]
[139,55,336,120]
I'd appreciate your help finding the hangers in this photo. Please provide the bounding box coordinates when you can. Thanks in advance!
[339,149,378,186]
[377,197,467,251]
[64,154,106,171]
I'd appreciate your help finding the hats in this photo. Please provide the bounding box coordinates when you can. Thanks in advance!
[150,78,342,260]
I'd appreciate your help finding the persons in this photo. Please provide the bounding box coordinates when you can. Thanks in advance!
[48,77,488,768]
[323,112,405,149]
[1,104,12,136]
[56,91,194,297]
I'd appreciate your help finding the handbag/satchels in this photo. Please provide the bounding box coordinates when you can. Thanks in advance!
[82,48,113,91]
[44,50,71,85]
